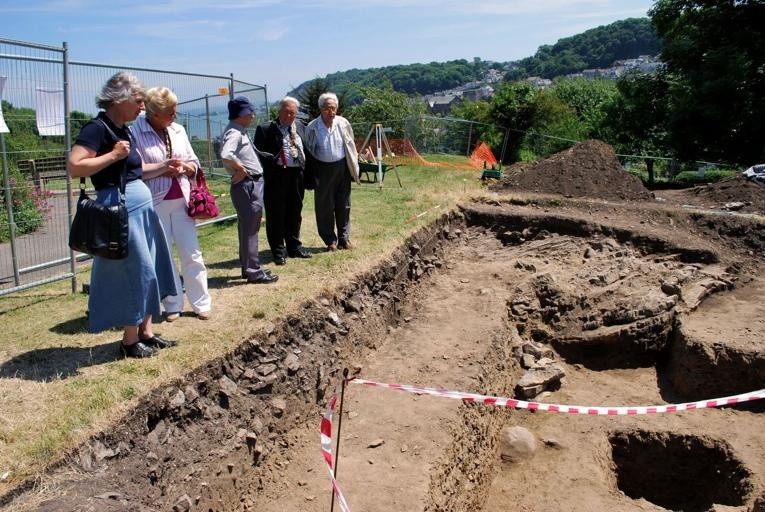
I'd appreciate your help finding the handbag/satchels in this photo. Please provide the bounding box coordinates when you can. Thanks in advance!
[185,160,219,219]
[69,194,128,259]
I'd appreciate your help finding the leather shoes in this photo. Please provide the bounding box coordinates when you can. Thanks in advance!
[121,335,176,359]
[242,268,278,284]
[274,247,312,265]
[326,240,356,251]
[198,311,212,320]
[166,312,180,322]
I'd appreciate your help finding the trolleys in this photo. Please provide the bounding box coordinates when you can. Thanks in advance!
[479,158,503,181]
[355,160,403,184]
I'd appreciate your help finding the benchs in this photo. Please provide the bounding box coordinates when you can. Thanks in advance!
[16,155,67,197]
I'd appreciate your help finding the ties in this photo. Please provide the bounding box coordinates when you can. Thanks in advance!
[288,127,305,170]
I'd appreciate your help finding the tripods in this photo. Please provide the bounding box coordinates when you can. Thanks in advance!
[358,124,402,191]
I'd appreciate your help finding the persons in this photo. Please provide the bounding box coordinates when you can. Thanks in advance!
[253,96,313,266]
[218,96,279,285]
[304,92,362,252]
[130,87,214,321]
[67,70,179,359]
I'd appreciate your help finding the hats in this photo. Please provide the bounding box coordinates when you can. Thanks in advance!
[228,97,253,119]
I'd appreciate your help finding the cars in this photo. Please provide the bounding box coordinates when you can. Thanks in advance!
[741,164,765,180]
